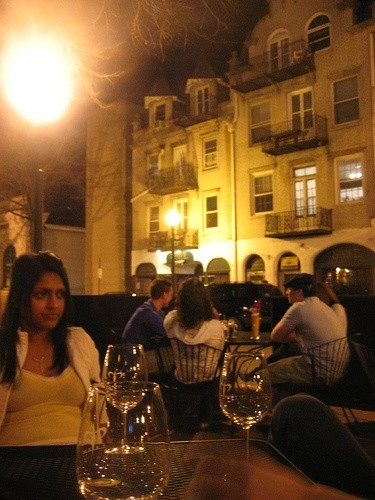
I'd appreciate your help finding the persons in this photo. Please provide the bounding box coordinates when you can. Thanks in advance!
[120,280,174,375]
[259,274,349,384]
[0,248,110,447]
[163,280,228,383]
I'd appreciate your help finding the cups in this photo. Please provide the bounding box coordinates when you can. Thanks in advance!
[73,381,173,500]
[222,319,238,341]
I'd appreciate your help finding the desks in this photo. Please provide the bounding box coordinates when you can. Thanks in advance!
[0,437,324,500]
[225,331,274,353]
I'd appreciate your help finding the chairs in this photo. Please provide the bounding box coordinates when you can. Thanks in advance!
[110,328,234,438]
[273,332,363,430]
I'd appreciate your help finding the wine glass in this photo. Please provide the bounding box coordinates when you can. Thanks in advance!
[101,344,148,455]
[218,351,272,445]
